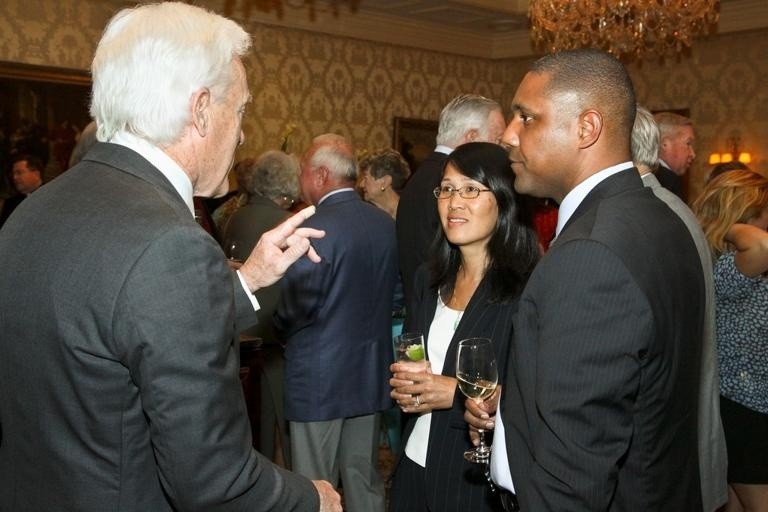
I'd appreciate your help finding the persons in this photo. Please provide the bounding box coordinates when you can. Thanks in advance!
[1,48,768,511]
[1,0,343,512]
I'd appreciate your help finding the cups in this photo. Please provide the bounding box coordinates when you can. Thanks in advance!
[391,331,429,414]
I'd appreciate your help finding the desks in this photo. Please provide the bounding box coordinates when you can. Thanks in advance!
[236,333,266,455]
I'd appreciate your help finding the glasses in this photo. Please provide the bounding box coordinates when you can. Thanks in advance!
[433,185,489,199]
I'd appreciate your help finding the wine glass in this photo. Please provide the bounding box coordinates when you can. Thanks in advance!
[454,337,500,464]
[223,237,245,266]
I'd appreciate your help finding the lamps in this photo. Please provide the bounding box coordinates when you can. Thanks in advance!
[525,0,721,60]
[706,128,752,166]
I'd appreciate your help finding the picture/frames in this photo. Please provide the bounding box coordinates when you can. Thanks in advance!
[1,62,100,216]
[392,116,440,173]
